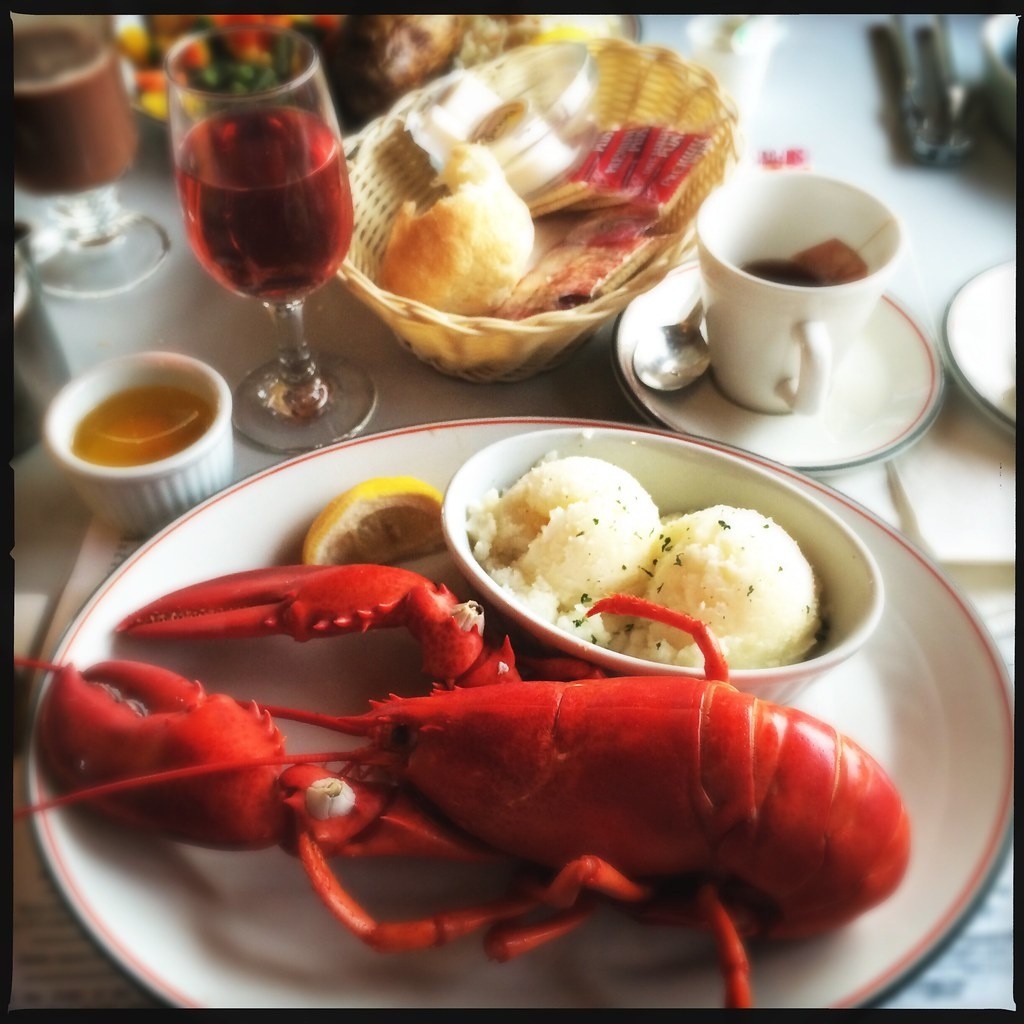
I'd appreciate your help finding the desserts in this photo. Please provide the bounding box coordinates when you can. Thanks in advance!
[440,428,885,706]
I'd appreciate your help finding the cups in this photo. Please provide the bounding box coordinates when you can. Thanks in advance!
[693,171,907,414]
[981,11,1016,152]
[40,352,234,543]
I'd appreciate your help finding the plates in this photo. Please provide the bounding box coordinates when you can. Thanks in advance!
[24,416,1013,1010]
[610,256,940,476]
[943,262,1015,436]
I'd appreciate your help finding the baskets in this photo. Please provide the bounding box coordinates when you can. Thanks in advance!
[322,26,741,384]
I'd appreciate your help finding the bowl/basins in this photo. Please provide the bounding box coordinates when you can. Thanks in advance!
[440,424,888,716]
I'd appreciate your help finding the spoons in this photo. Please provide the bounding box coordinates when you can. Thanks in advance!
[634,294,711,392]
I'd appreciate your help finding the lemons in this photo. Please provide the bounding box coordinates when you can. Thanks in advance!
[303,476,444,566]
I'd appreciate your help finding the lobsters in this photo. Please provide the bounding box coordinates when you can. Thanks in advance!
[12,562,913,1011]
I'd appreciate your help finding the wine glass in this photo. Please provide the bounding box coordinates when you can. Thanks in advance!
[161,23,380,455]
[13,15,173,300]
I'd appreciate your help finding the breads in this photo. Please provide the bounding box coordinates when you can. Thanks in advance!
[381,143,533,317]
